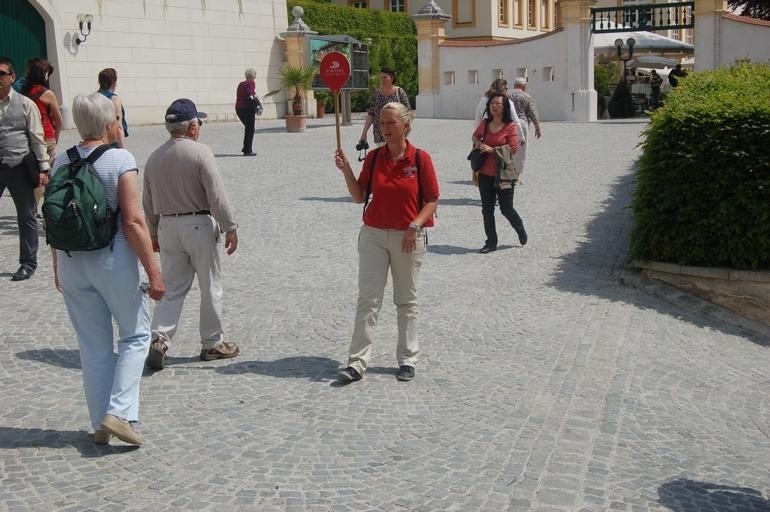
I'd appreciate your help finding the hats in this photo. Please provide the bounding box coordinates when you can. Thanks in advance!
[165,98,207,122]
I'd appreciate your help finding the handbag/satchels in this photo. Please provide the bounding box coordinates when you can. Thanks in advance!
[467,140,488,171]
[248,97,263,116]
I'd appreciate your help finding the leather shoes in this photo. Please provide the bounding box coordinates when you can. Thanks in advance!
[243,150,256,156]
[480,245,497,254]
[516,226,527,245]
[337,366,362,382]
[397,366,415,381]
[12,265,33,281]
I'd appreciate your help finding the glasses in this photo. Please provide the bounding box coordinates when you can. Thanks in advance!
[0,70,11,80]
[197,119,203,126]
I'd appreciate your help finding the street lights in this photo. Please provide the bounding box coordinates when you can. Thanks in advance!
[614,35,638,120]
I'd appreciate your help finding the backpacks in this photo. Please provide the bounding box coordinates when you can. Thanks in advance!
[41,144,118,252]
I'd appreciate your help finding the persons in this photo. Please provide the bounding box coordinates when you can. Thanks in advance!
[360,68,412,148]
[508,77,541,187]
[235,67,263,156]
[142,98,240,370]
[668,64,688,88]
[97,67,130,149]
[334,102,441,382]
[11,65,54,92]
[472,93,527,255]
[475,79,525,206]
[22,57,63,168]
[0,57,51,280]
[650,70,663,108]
[46,93,167,445]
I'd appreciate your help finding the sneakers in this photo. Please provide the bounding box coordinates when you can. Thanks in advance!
[148,337,168,369]
[199,342,240,361]
[93,429,111,444]
[100,415,143,445]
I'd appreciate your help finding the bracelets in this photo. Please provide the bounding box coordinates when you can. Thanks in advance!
[409,222,420,231]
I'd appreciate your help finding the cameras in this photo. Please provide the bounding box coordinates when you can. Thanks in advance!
[356,140,369,151]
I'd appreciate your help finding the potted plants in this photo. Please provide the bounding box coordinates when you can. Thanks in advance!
[313,89,329,119]
[259,63,321,132]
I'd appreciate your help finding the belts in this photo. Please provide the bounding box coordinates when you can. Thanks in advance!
[166,210,210,215]
